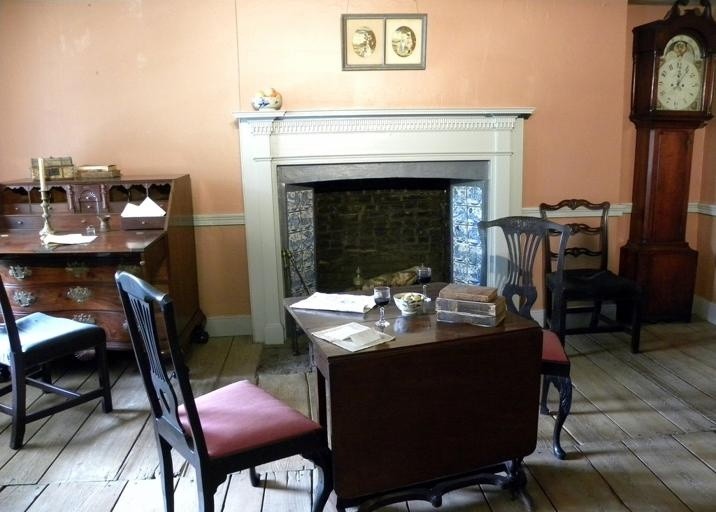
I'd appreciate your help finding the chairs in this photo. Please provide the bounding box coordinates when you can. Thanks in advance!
[540,199,644,354]
[0,276,112,449]
[477,216,572,459]
[114,272,332,511]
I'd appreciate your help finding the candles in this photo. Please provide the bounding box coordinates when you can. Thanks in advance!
[38,159,46,190]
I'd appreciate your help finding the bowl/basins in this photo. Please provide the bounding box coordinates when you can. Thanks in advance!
[252,95,281,112]
[393,316,417,333]
[393,292,425,315]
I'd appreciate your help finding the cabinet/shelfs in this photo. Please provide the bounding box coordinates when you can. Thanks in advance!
[0,173,211,364]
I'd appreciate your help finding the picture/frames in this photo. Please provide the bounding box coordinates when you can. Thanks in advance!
[342,14,427,70]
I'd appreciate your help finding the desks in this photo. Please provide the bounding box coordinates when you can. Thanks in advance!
[282,283,543,512]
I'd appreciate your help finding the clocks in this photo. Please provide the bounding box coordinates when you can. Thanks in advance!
[628,0,716,128]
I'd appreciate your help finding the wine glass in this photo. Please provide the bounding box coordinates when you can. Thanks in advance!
[417,267,431,297]
[375,328,390,350]
[373,287,390,327]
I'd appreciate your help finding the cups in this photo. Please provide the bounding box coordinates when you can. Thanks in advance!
[86,225,95,236]
[96,213,112,230]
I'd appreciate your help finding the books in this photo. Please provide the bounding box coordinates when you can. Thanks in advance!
[435,283,507,327]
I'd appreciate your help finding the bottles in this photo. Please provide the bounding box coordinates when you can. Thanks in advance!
[353,266,363,291]
